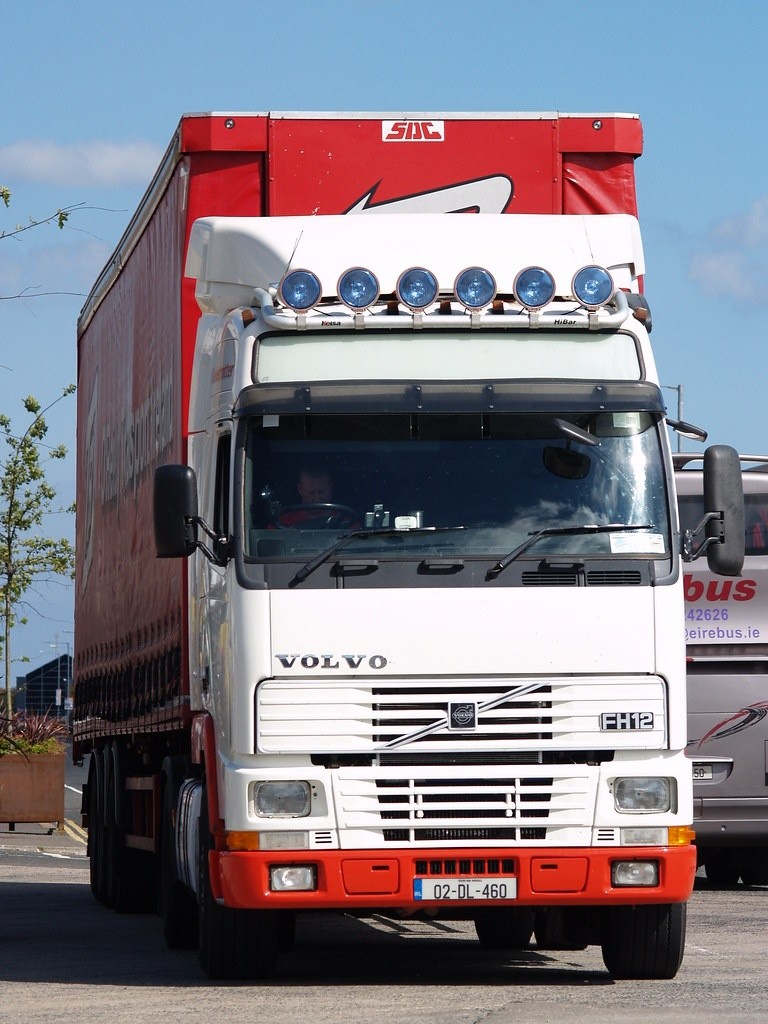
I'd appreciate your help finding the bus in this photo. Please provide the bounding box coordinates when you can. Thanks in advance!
[666,454,768,891]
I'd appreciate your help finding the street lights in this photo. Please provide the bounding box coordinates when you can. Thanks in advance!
[39,639,72,741]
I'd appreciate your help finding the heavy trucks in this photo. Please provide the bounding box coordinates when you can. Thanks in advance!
[70,111,748,983]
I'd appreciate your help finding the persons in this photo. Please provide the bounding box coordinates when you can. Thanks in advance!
[268,462,360,530]
